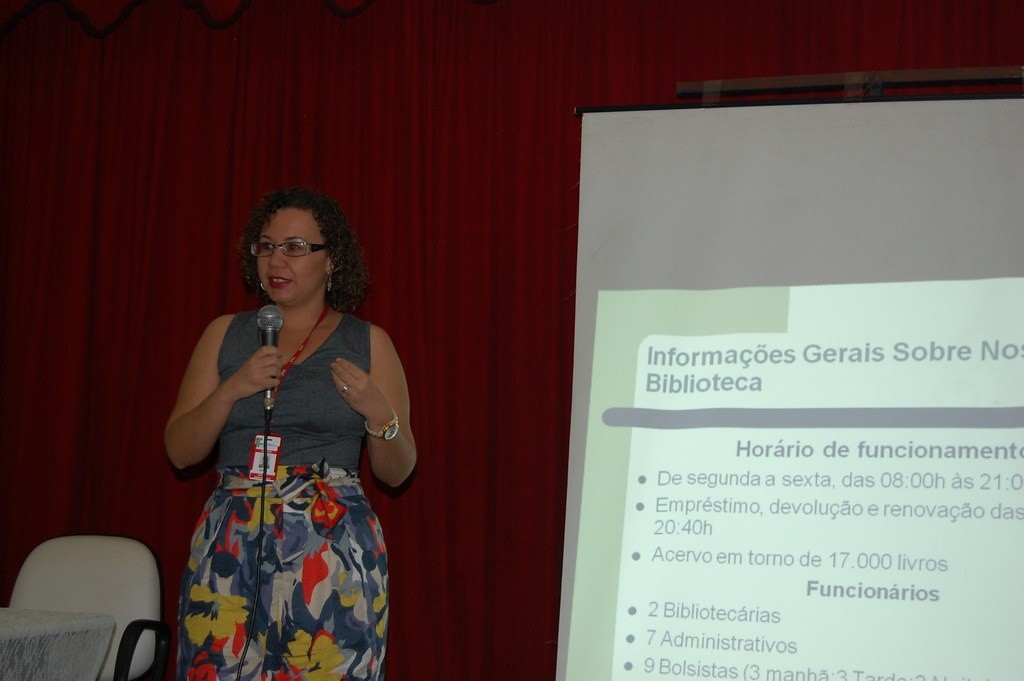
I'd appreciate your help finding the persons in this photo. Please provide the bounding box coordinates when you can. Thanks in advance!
[163,186,417,680]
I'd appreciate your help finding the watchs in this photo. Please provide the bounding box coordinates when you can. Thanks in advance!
[365,409,399,441]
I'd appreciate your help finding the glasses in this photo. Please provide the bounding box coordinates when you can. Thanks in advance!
[248,240,329,257]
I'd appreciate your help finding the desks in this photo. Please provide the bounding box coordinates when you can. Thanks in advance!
[0,608,114,680]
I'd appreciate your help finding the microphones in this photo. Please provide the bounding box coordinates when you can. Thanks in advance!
[256,305,285,421]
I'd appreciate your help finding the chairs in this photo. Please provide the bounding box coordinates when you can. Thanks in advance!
[12,534,171,681]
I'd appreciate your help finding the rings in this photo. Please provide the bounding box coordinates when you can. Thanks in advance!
[343,386,350,392]
